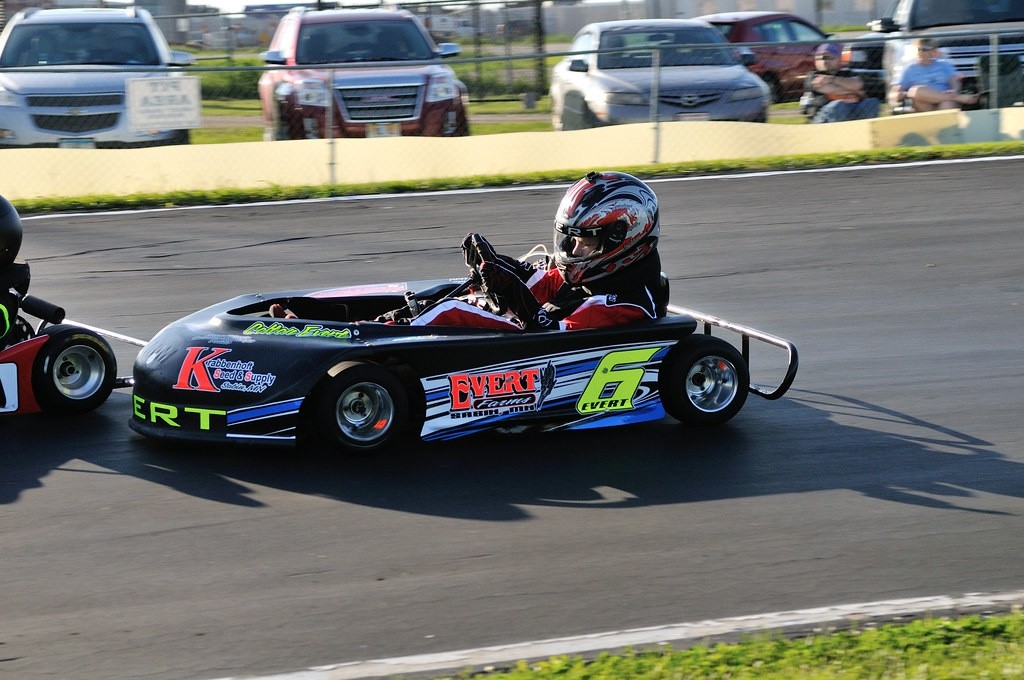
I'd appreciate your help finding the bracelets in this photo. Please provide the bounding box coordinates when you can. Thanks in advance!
[830,75,835,84]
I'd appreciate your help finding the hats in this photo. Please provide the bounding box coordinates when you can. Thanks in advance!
[815,42,841,55]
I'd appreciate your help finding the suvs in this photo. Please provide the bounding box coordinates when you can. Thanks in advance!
[255,3,471,140]
[0,5,193,150]
[865,0,1024,114]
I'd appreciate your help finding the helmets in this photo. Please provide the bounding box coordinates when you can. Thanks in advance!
[0,194,23,275]
[553,171,660,287]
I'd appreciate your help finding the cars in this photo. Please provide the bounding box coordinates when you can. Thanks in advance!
[549,18,774,133]
[697,11,836,102]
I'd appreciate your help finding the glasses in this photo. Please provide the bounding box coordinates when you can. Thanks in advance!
[815,56,836,60]
[918,48,931,51]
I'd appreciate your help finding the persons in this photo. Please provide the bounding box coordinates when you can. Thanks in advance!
[891,36,978,113]
[804,42,878,124]
[0,197,25,353]
[272,173,667,334]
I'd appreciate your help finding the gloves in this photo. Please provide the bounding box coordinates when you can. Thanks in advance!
[460,233,520,274]
[479,261,539,323]
